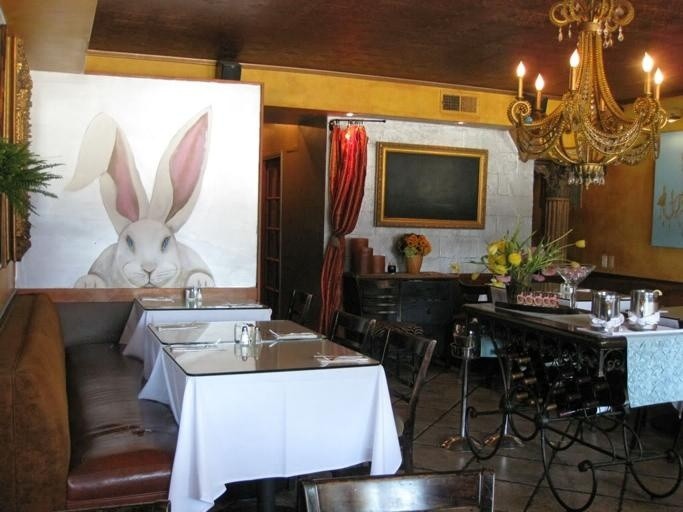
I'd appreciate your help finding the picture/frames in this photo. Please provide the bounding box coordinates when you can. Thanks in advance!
[374,140,489,230]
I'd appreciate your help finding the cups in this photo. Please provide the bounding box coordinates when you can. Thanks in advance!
[559,282,573,300]
[598,253,616,274]
[387,262,396,274]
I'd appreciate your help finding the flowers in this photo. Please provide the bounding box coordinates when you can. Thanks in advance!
[395,231,433,264]
[450,220,587,282]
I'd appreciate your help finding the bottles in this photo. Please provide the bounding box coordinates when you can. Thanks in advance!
[251,326,262,345]
[189,289,202,300]
[499,348,616,423]
[591,291,620,333]
[252,344,262,361]
[240,327,250,346]
[626,289,660,331]
[188,301,202,308]
[240,345,250,362]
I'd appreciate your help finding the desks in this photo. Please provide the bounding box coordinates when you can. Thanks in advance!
[117,297,404,512]
[457,303,683,512]
[341,271,492,370]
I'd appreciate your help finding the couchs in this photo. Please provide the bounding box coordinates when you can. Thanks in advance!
[2,292,182,511]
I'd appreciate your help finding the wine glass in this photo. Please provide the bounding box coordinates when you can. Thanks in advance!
[556,263,595,310]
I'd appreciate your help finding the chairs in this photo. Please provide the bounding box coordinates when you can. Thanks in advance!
[280,287,496,512]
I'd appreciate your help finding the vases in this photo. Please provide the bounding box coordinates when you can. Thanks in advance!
[506,282,531,304]
[405,255,423,274]
